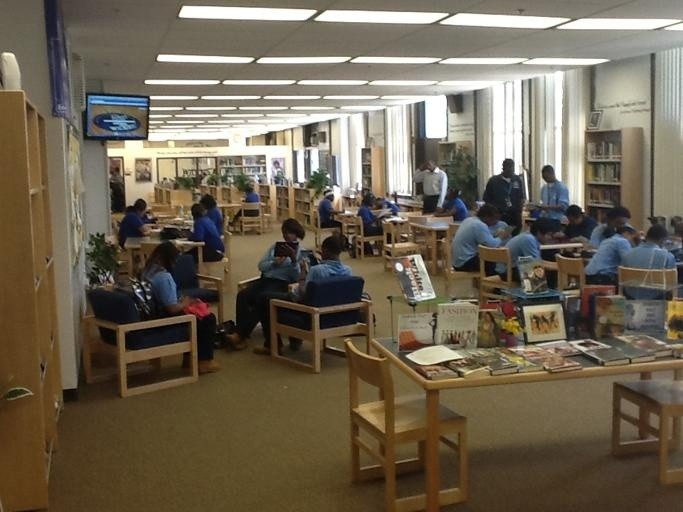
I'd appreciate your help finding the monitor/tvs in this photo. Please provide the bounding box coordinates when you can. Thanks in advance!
[85,93,150,142]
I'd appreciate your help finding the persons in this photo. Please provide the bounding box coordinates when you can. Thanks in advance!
[412,157,448,216]
[433,158,683,300]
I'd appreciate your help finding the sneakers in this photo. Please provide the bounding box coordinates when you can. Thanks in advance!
[289,342,302,353]
[252,345,283,356]
[198,360,222,374]
[226,331,240,346]
[234,340,249,352]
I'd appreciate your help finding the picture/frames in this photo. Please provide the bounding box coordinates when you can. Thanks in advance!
[521,302,568,345]
[155,156,217,183]
[586,109,603,130]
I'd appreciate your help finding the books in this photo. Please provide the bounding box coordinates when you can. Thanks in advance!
[397,295,683,381]
[587,140,621,224]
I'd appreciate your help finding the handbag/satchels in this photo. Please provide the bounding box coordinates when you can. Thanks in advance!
[212,320,237,349]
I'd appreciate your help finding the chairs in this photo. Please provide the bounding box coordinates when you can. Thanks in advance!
[270,275,375,373]
[82,289,198,398]
[343,337,469,512]
[288,182,680,306]
[81,182,298,331]
[612,300,680,487]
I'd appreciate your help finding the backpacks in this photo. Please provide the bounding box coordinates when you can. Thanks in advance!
[128,274,155,319]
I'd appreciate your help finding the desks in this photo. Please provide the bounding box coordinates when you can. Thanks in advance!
[369,336,682,511]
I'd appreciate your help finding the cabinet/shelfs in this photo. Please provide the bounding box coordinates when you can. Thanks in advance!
[217,154,268,176]
[0,90,67,511]
[436,140,475,208]
[44,118,92,393]
[581,126,642,232]
[361,146,385,199]
